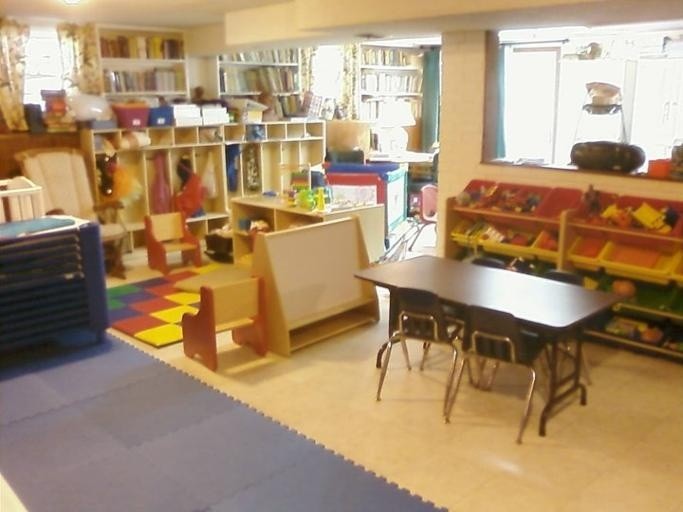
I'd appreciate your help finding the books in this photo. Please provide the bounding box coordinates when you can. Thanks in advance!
[362,97,421,118]
[361,71,423,93]
[100,34,184,59]
[172,104,230,127]
[220,66,295,92]
[103,69,183,92]
[219,48,298,64]
[362,48,408,66]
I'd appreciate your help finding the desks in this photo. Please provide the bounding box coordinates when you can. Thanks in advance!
[354,254,623,436]
[366,150,439,167]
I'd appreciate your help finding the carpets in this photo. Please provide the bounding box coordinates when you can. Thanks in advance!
[106,270,254,348]
[0,335,451,511]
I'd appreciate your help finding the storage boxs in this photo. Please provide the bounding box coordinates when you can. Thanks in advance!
[113,107,148,127]
[150,107,172,126]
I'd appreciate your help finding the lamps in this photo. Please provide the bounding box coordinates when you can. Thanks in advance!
[376,101,416,151]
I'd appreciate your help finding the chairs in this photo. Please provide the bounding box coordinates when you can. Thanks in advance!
[420,258,506,385]
[330,149,364,165]
[487,270,593,386]
[182,272,267,372]
[12,148,129,281]
[445,304,550,445]
[409,184,439,251]
[376,287,473,415]
[143,211,202,276]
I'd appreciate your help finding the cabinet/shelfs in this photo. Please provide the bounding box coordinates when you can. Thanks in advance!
[445,178,683,364]
[93,26,192,107]
[357,44,423,122]
[231,195,385,264]
[82,119,329,251]
[217,48,303,109]
